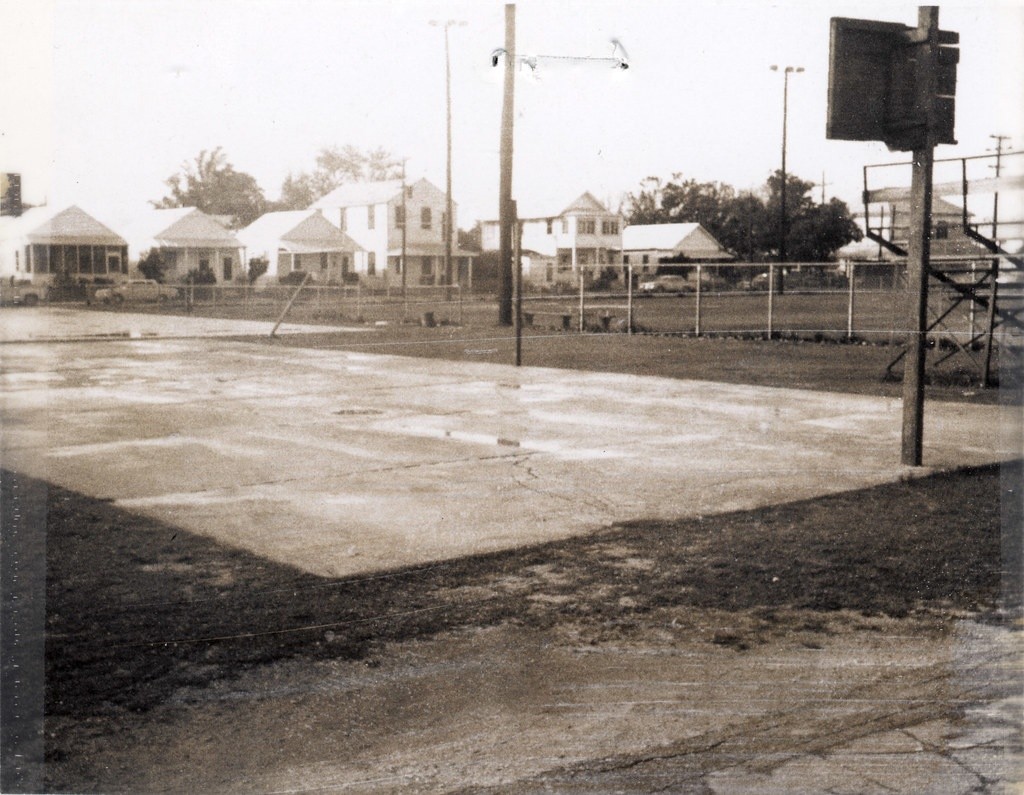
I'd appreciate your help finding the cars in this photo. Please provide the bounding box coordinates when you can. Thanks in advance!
[639,274,689,295]
[93,279,179,305]
[0,275,50,306]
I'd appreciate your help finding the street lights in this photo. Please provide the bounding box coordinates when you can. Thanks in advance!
[771,64,803,296]
[428,16,467,302]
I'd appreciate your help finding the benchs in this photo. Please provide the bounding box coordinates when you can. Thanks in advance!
[520,309,572,330]
[576,304,634,330]
